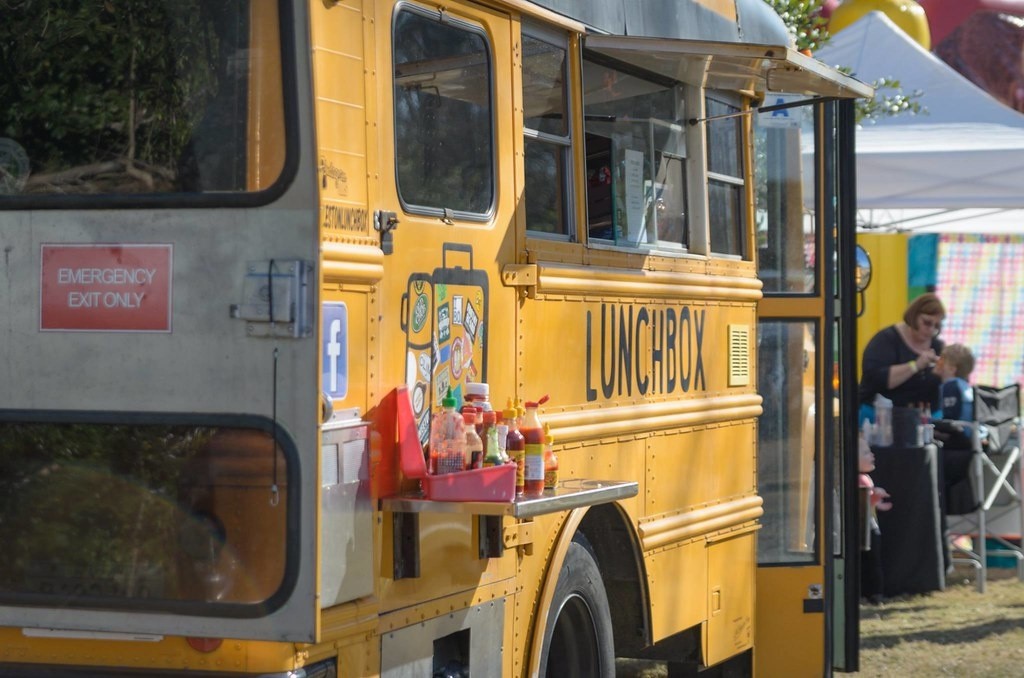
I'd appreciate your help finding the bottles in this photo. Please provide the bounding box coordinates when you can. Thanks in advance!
[874,393,893,447]
[925,402,932,424]
[917,402,927,424]
[429,382,559,494]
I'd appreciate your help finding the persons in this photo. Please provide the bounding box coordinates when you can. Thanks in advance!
[930,344,976,441]
[858,293,945,414]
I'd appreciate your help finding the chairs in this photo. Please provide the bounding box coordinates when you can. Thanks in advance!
[934,383,1024,596]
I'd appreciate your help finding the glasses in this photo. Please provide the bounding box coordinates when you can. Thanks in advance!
[918,315,942,329]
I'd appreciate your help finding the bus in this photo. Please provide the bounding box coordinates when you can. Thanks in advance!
[2,0,871,678]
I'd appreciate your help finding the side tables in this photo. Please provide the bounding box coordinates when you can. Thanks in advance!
[871,442,945,601]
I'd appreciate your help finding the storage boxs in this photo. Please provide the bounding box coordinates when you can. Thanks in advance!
[396,388,518,501]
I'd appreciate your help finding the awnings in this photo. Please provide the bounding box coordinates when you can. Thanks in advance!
[804,11,1024,209]
